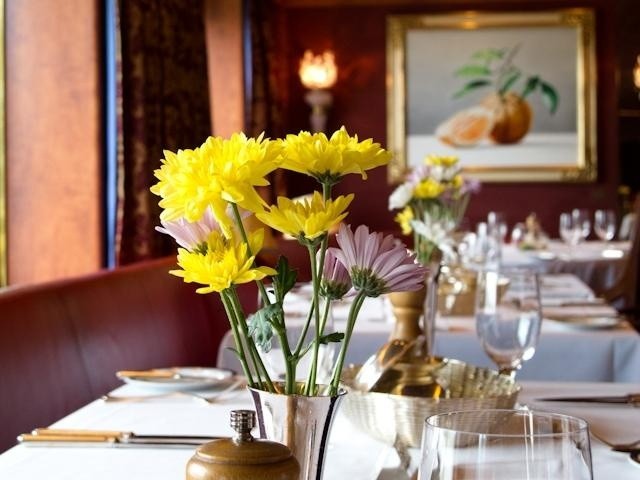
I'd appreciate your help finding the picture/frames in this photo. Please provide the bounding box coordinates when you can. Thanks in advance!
[384,6,599,187]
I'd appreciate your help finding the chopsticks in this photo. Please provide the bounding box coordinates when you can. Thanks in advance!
[15,428,233,450]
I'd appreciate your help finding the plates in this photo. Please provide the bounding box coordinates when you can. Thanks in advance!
[115,364,236,392]
[328,354,523,451]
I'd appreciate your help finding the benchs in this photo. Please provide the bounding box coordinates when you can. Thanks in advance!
[1,244,306,450]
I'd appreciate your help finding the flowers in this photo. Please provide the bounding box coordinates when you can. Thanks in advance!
[151,130,426,395]
[388,153,483,241]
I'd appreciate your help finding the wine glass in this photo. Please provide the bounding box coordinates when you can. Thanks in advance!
[560,207,617,256]
[416,410,593,479]
[474,221,541,379]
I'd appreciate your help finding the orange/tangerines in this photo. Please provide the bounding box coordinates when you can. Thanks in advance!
[435,106,496,147]
[481,91,531,144]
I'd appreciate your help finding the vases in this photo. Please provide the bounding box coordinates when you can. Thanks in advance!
[244,380,351,479]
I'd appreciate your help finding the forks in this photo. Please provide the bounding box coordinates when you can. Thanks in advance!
[103,382,246,404]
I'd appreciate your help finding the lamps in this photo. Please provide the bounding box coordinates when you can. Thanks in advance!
[299,48,338,134]
